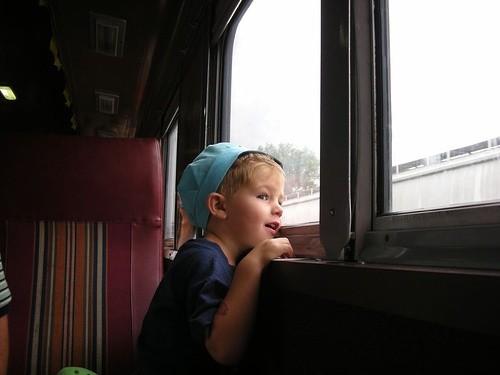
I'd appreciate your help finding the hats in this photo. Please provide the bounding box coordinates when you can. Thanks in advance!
[177,141,283,230]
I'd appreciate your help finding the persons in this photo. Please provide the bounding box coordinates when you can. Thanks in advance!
[116,144,293,375]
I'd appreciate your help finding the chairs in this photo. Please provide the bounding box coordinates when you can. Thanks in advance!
[0,132,165,375]
